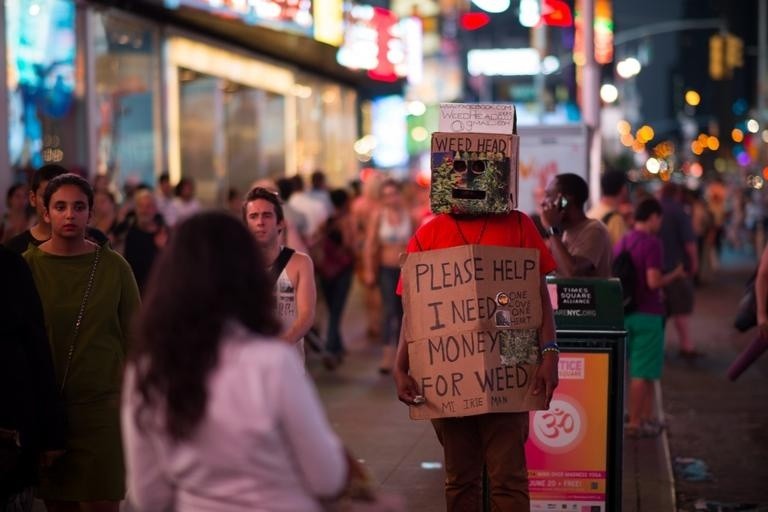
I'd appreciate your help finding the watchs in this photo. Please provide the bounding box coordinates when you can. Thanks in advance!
[546,226,562,235]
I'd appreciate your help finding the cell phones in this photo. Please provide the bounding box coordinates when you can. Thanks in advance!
[553,196,568,213]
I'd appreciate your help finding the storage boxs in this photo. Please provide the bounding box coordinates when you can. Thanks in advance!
[432,131,519,214]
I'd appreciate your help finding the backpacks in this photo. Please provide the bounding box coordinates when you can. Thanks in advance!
[611,235,645,316]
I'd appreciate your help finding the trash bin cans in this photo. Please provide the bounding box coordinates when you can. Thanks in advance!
[525,276,628,512]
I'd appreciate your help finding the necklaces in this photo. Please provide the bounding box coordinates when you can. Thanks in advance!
[452,219,487,245]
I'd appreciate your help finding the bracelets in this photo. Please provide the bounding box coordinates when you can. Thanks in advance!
[540,348,560,355]
[541,342,558,348]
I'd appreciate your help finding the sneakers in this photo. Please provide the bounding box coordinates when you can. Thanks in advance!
[624,413,660,439]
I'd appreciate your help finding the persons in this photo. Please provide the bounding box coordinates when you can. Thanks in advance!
[633,179,768,355]
[611,197,687,438]
[1,163,435,374]
[21,173,143,512]
[585,169,628,244]
[0,244,57,512]
[393,209,558,512]
[119,210,349,512]
[538,174,613,280]
